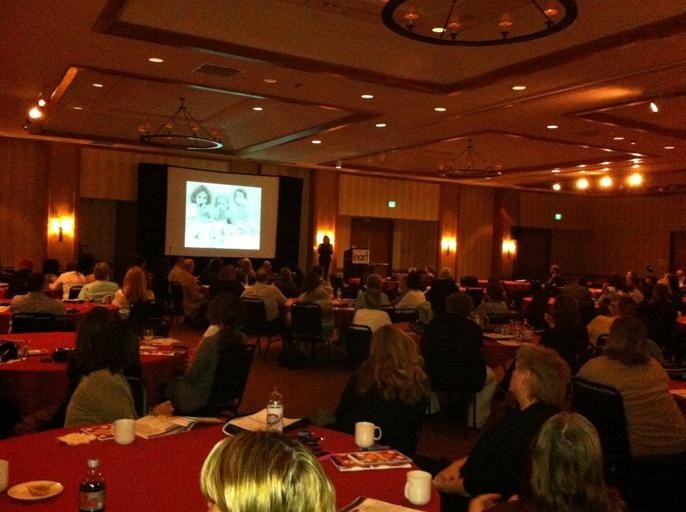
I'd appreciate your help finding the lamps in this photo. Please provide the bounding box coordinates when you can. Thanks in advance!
[650,100,666,112]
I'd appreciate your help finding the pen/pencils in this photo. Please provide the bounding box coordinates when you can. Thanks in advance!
[359,446,391,451]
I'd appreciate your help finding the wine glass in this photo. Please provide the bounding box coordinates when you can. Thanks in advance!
[142,329,154,356]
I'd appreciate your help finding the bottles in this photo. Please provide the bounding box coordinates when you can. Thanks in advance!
[336,288,342,299]
[267,405,283,433]
[80,459,104,512]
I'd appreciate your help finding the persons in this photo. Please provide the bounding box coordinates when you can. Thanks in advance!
[186,184,260,240]
[315,234,336,279]
[1,256,686,512]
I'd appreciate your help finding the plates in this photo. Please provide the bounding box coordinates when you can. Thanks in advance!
[8,480,64,501]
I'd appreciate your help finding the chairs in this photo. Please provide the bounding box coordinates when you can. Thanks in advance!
[0,280,686,512]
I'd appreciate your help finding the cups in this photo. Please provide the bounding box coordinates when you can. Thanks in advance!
[0,460,8,493]
[354,422,382,448]
[404,470,433,506]
[111,419,135,445]
